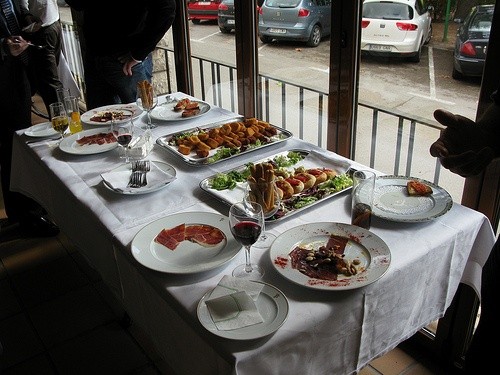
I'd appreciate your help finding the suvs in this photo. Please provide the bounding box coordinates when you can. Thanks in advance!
[187,0,222,24]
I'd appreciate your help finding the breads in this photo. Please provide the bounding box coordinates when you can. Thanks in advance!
[275,168,337,198]
[406,182,432,195]
[178,118,278,158]
[174,98,201,116]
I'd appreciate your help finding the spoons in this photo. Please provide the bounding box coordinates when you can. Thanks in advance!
[14,39,47,49]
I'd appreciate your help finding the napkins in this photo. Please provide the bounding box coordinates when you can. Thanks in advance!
[100,161,174,194]
[205,275,265,332]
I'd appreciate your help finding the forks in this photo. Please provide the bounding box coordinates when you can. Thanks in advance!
[129,160,151,189]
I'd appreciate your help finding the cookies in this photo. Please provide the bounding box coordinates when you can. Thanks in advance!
[248,162,274,211]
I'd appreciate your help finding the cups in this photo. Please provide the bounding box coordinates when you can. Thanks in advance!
[63,96,84,133]
[352,170,376,230]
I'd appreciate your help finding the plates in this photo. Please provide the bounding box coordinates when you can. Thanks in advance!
[350,175,455,223]
[80,103,144,126]
[148,99,211,121]
[25,121,70,137]
[270,222,391,291]
[196,281,289,341]
[130,211,240,274]
[58,126,122,156]
[101,159,176,194]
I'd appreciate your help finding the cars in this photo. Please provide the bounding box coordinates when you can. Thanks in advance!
[360,0,433,64]
[451,4,495,81]
[259,0,332,48]
[217,0,236,34]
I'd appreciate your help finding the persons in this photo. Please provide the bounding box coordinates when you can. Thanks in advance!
[430,84,500,375]
[0,0,62,236]
[67,0,175,113]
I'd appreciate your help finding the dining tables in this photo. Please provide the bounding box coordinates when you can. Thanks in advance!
[9,91,496,375]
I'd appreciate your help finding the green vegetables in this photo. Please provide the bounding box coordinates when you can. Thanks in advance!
[206,138,306,190]
[288,173,353,208]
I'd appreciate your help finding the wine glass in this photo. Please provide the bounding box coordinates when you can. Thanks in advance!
[244,178,281,248]
[136,85,158,130]
[49,102,68,140]
[228,202,265,280]
[112,116,134,160]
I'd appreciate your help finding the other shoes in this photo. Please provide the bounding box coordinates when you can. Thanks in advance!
[7,217,60,237]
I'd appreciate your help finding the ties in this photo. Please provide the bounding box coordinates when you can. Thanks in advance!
[0,0,32,66]
